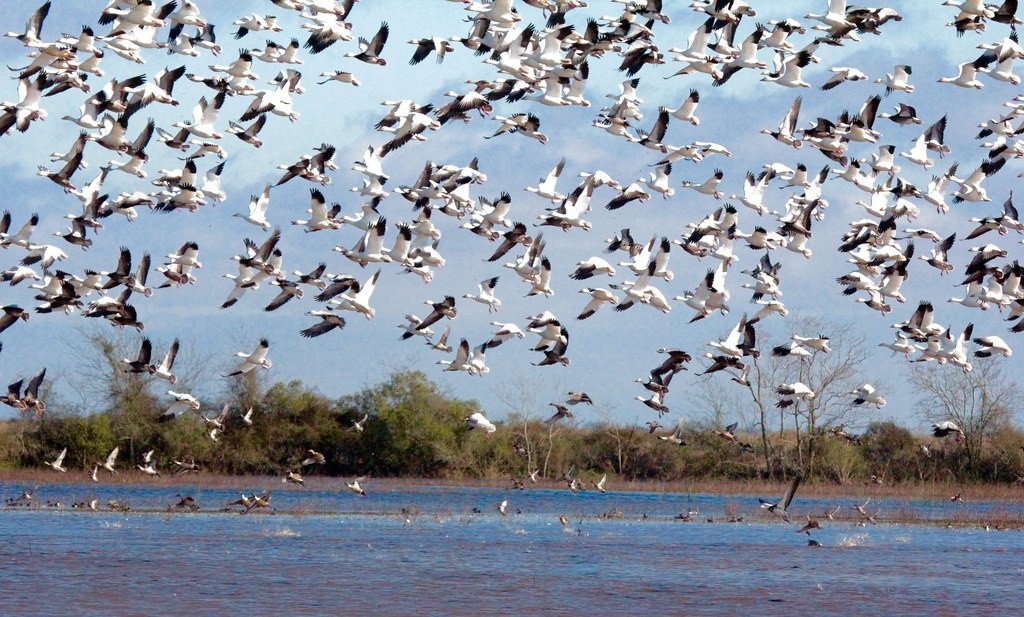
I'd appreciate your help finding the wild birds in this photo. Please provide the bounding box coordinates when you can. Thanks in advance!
[0,0,1023,546]
